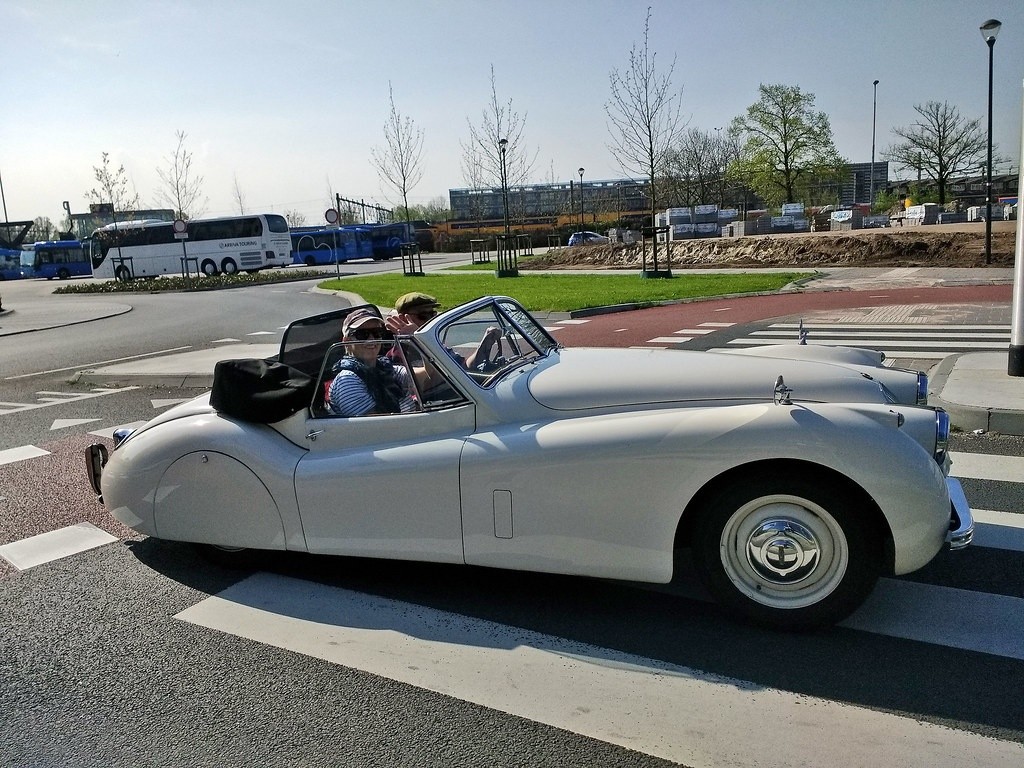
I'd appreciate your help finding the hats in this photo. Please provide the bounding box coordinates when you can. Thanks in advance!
[395,292,441,313]
[342,309,386,338]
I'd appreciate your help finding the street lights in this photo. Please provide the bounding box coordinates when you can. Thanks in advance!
[616,183,620,229]
[577,167,584,245]
[869,80,879,202]
[979,19,1003,265]
[498,138,512,269]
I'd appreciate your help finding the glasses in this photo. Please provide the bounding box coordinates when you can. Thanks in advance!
[405,310,438,322]
[350,326,386,341]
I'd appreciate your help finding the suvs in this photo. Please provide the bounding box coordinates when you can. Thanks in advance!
[568,232,611,246]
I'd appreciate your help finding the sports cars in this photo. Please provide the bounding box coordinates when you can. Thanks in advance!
[84,294,973,631]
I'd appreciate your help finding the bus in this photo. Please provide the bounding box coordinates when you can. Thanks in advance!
[80,213,295,281]
[289,228,357,267]
[349,228,373,259]
[0,248,22,281]
[18,241,93,280]
[360,223,417,260]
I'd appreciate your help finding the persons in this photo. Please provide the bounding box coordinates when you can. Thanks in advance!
[380,292,502,402]
[328,309,440,417]
[810,215,816,233]
